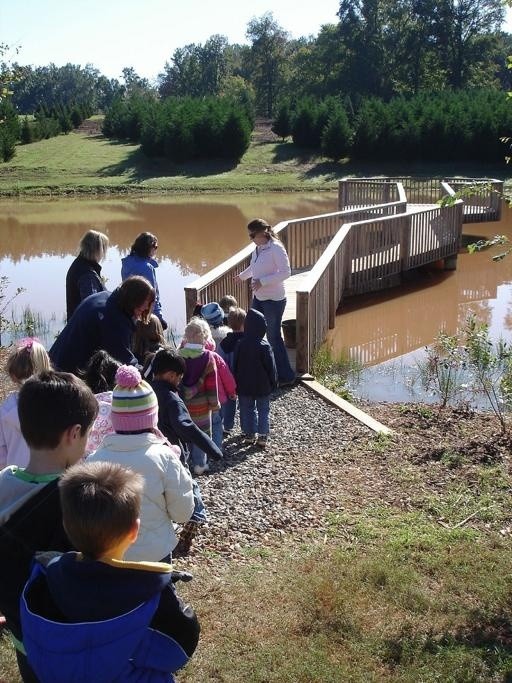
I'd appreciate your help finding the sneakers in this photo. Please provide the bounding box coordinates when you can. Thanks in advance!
[244,431,257,446]
[256,434,269,447]
[194,463,211,475]
[180,519,198,542]
[223,427,235,434]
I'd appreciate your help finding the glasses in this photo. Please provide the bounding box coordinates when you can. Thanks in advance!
[247,231,264,238]
[150,243,158,247]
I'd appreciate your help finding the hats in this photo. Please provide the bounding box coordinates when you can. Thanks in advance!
[201,301,226,327]
[112,364,159,432]
